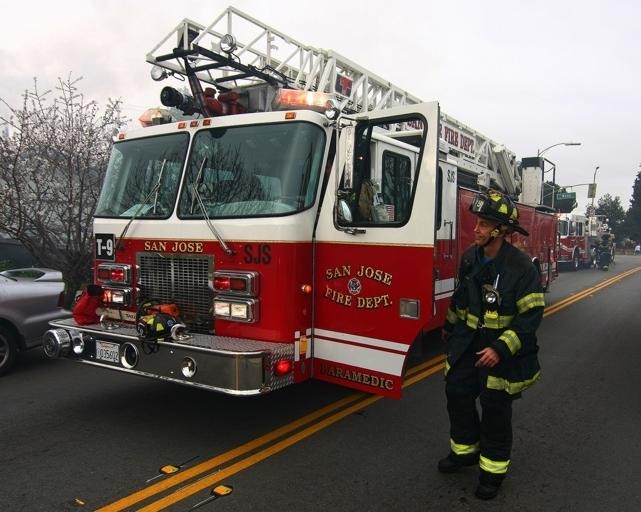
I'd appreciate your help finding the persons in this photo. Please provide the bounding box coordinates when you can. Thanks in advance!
[323,150,371,223]
[437,188,546,500]
[598,233,614,271]
[635,243,641,256]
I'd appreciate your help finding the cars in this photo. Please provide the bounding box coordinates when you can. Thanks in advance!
[0,268,72,377]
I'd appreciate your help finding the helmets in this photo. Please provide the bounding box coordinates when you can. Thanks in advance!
[468,187,531,237]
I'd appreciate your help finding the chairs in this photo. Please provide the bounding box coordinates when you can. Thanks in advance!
[214,179,264,203]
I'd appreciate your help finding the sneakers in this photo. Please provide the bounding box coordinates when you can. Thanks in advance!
[436,452,479,473]
[472,479,501,501]
